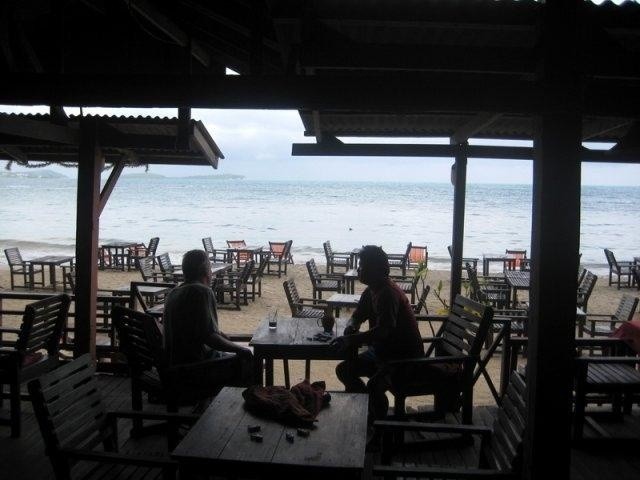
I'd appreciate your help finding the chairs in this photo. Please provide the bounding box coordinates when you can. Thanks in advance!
[388,263,431,316]
[324,240,355,273]
[110,306,245,427]
[3,247,46,290]
[448,246,531,357]
[577,265,598,313]
[307,259,346,305]
[138,252,271,311]
[388,242,411,277]
[0,293,71,437]
[202,239,294,277]
[284,279,324,319]
[61,263,76,295]
[128,237,160,271]
[27,352,202,480]
[605,248,639,290]
[575,294,640,416]
[368,294,495,425]
[368,370,535,480]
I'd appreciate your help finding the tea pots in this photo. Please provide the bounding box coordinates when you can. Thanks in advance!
[317,305,337,332]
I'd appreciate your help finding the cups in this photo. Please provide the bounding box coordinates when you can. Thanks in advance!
[268,308,279,329]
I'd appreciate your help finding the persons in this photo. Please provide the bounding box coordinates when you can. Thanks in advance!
[329,245,426,393]
[160,249,254,393]
